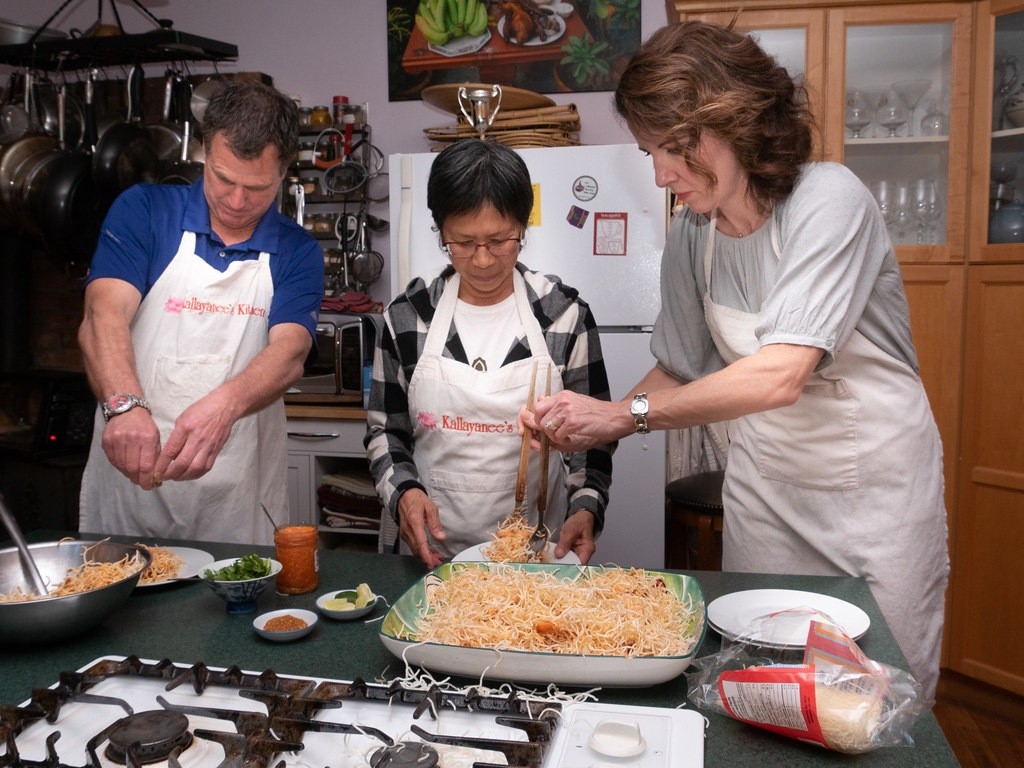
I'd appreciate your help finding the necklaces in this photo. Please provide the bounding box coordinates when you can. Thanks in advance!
[717,210,763,237]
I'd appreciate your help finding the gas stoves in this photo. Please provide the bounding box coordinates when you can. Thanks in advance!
[0,653,704,768]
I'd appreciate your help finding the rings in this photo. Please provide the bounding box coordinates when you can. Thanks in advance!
[546,420,559,431]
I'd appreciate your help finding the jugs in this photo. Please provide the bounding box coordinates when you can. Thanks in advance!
[991,49,1020,132]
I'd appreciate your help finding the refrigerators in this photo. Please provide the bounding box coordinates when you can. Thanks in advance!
[388,144,672,570]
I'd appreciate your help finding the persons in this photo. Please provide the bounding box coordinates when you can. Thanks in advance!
[363,142,617,570]
[78,84,324,546]
[519,20,949,709]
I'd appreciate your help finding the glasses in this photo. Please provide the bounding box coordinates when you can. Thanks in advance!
[437,224,523,258]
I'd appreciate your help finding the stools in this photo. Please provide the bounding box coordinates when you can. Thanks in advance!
[666,471,724,571]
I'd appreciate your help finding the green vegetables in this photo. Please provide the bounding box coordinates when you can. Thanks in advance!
[202,553,271,582]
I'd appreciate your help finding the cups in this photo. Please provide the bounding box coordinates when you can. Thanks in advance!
[536,5,557,36]
[273,523,319,596]
[991,163,1017,183]
[989,185,1015,222]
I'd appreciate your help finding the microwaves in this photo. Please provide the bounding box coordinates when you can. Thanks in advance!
[284,312,376,397]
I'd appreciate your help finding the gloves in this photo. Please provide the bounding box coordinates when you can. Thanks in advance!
[318,290,375,312]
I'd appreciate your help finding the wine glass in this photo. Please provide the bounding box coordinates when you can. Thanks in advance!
[844,79,949,140]
[869,179,944,246]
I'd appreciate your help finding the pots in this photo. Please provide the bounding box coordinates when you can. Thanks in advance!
[0,64,205,246]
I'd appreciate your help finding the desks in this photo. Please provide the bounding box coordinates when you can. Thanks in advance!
[0,531,963,768]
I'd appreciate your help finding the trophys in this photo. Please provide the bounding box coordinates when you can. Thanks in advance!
[458,85,502,140]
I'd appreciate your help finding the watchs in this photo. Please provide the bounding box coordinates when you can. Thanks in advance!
[631,392,650,451]
[102,393,153,423]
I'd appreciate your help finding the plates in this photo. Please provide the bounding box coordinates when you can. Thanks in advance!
[497,12,566,46]
[378,561,707,687]
[428,27,492,57]
[451,538,582,565]
[530,0,561,6]
[706,589,871,650]
[134,546,214,588]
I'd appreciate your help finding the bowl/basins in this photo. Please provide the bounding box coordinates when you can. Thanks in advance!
[315,589,378,620]
[555,3,574,18]
[198,557,283,614]
[0,541,153,645]
[253,609,318,642]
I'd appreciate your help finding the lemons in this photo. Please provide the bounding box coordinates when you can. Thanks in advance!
[324,583,376,612]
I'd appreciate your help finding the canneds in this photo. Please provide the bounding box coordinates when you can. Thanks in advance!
[273,523,320,595]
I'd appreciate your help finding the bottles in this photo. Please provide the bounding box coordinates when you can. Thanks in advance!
[287,134,367,296]
[291,96,362,126]
[1004,83,1024,128]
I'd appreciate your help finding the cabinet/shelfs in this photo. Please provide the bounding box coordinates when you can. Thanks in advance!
[665,0,1024,723]
[276,123,371,293]
[281,391,367,529]
